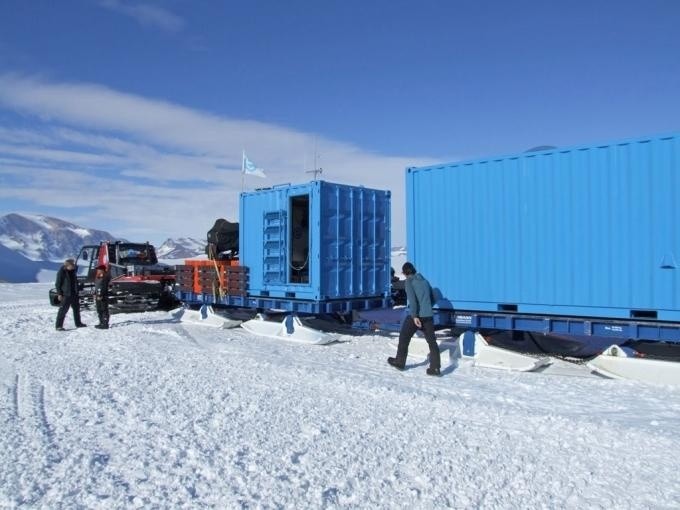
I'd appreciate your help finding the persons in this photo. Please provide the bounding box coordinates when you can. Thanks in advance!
[139,252,146,258]
[92,265,111,330]
[55,259,87,330]
[387,261,441,377]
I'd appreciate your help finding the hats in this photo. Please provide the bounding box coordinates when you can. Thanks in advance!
[96,265,106,270]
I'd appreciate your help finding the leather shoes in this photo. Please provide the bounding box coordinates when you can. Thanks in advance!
[426,368,440,375]
[56,327,64,331]
[95,325,109,328]
[77,324,86,327]
[388,357,406,370]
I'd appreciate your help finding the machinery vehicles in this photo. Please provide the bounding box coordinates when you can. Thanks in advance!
[48,235,179,313]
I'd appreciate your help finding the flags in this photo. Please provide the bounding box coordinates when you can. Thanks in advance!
[242,150,267,178]
